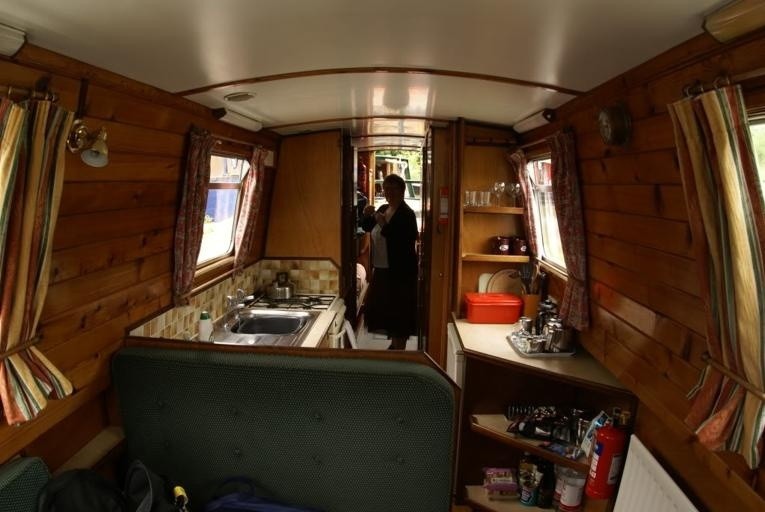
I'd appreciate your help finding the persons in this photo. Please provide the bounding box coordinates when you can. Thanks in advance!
[360,172,418,350]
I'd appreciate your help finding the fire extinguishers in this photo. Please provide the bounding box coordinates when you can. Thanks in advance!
[584,407,630,500]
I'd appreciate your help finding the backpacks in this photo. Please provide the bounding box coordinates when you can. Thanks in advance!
[34,457,188,512]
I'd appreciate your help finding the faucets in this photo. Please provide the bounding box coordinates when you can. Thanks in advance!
[221,293,235,328]
[233,288,246,318]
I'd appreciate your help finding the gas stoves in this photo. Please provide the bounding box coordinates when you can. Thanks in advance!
[252,291,336,316]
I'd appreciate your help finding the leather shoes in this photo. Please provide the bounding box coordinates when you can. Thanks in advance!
[387,339,405,350]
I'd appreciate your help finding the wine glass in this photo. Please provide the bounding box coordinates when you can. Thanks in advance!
[493,181,521,207]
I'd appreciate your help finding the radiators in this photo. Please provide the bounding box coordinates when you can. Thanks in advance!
[611,434,699,512]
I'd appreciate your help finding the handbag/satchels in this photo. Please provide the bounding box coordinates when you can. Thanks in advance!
[204,476,309,511]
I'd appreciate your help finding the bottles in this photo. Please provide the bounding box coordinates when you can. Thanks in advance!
[552,466,586,511]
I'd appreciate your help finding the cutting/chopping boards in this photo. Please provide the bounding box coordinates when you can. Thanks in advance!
[488,269,524,295]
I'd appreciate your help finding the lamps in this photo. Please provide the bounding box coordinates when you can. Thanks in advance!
[66,119,110,169]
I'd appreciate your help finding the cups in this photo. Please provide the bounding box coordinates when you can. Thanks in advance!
[465,190,492,208]
[522,294,542,316]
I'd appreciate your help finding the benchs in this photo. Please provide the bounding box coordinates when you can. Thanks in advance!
[109,336,462,512]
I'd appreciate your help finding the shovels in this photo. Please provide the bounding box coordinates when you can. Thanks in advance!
[519,263,539,295]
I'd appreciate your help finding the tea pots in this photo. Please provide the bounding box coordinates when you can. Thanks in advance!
[266,272,294,300]
[535,298,566,354]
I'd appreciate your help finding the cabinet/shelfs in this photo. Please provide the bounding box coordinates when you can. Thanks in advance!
[452,116,533,319]
[444,310,639,511]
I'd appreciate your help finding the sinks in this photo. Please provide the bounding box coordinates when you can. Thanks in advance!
[231,310,322,335]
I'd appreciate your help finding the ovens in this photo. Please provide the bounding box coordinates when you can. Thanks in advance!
[329,306,353,350]
